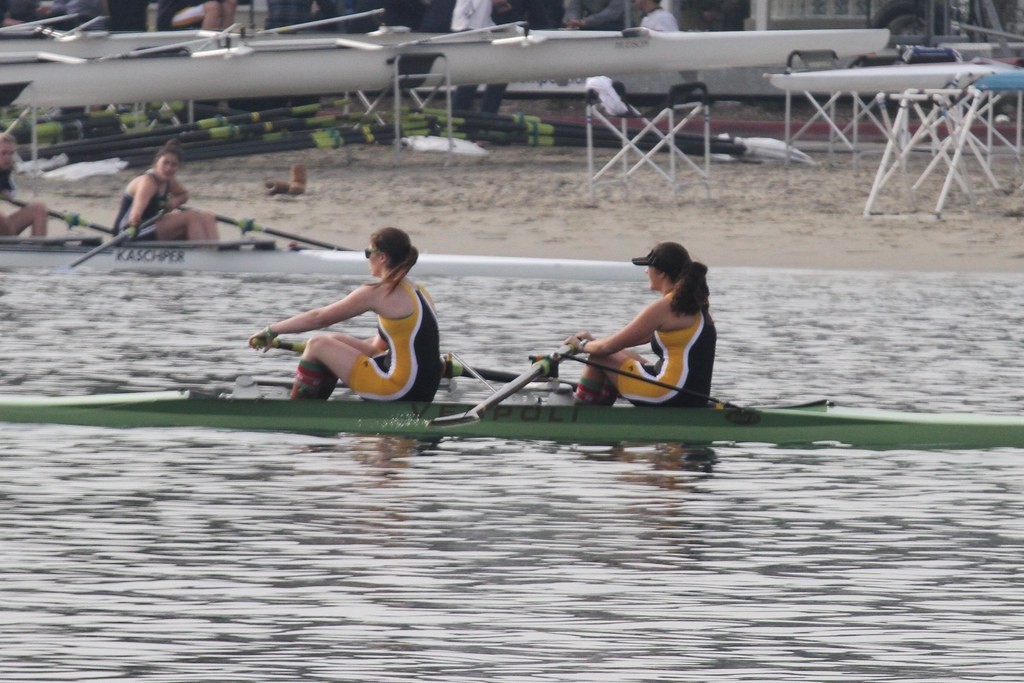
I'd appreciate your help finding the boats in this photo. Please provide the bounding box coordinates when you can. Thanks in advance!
[0,393,1024,442]
[768,59,1024,96]
[0,19,893,108]
[0,236,650,283]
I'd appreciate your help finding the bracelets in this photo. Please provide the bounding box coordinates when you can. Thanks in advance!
[579,339,588,351]
[264,327,277,339]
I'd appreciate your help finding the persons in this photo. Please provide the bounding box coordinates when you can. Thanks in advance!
[688,0,751,32]
[113,139,222,252]
[0,0,150,134]
[0,132,49,236]
[565,243,718,407]
[156,0,237,32]
[248,228,440,401]
[263,0,680,112]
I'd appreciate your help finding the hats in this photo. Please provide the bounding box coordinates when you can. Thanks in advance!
[631,249,680,275]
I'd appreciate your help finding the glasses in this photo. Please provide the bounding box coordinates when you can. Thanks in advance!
[365,247,385,258]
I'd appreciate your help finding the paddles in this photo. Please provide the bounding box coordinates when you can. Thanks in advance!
[0,192,113,234]
[250,336,578,393]
[178,205,354,251]
[66,207,169,270]
[424,333,588,429]
[1,98,818,183]
